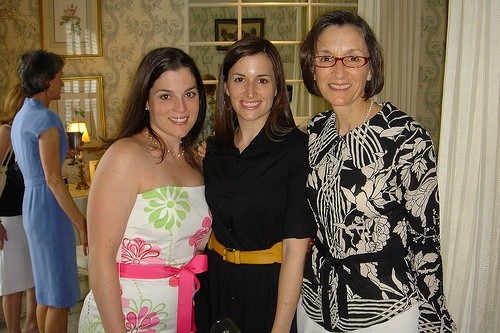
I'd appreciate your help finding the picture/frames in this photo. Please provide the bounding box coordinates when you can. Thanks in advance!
[38,0,104,58]
[214,18,264,52]
[56,75,106,153]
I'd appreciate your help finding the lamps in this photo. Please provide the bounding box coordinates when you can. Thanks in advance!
[67,122,92,192]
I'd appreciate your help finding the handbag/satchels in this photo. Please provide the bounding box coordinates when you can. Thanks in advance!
[0,124,14,195]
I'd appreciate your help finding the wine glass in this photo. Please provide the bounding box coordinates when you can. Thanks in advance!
[66,132,85,167]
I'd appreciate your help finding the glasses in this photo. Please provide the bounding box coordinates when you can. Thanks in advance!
[311,51,371,68]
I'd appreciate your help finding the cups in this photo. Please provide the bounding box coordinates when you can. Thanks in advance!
[210,318,242,333]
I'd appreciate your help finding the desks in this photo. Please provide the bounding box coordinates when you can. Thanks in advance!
[68,182,91,245]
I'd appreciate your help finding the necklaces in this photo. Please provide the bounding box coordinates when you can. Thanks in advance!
[144,128,185,157]
[363,99,374,123]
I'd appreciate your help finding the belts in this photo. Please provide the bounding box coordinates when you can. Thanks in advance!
[207,232,285,265]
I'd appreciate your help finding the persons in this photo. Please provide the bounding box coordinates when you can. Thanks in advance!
[77,47,214,333]
[11,50,88,333]
[0,82,38,333]
[297,10,458,333]
[194,36,312,333]
